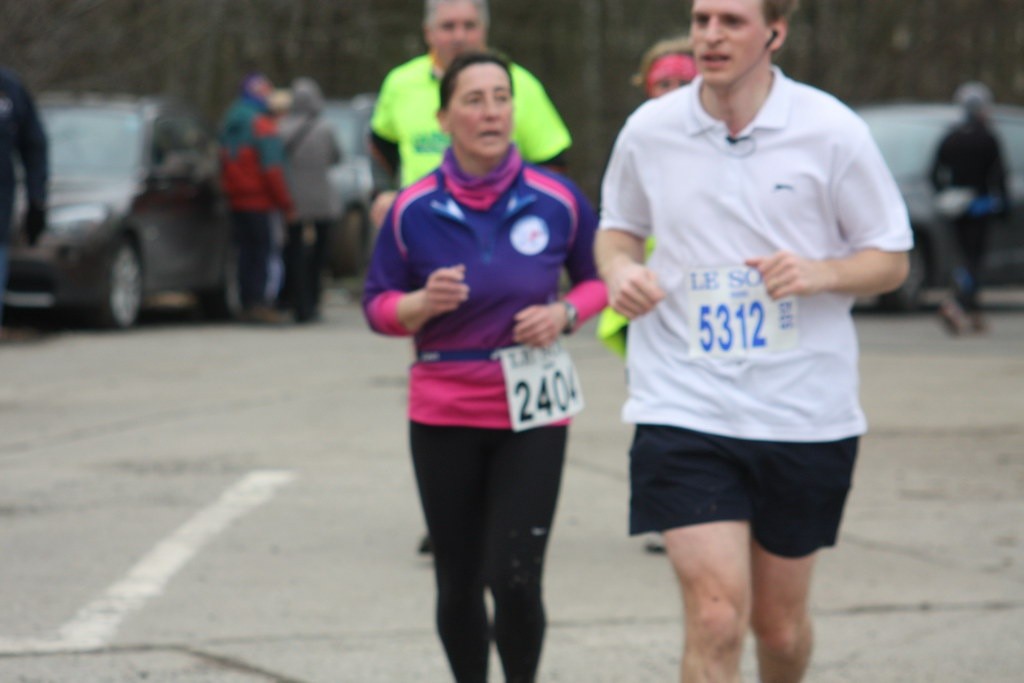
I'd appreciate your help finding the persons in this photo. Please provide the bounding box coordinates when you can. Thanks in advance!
[0,70,50,310]
[360,1,914,683]
[218,72,344,323]
[931,78,1009,334]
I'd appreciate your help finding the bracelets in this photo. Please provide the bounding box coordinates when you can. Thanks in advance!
[562,301,578,325]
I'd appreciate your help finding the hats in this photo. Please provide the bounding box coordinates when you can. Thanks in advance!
[291,79,323,113]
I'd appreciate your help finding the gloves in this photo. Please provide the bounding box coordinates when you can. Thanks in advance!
[22,208,48,247]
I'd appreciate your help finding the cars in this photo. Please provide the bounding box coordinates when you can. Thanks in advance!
[0,87,249,336]
[842,95,1024,317]
[297,88,395,289]
